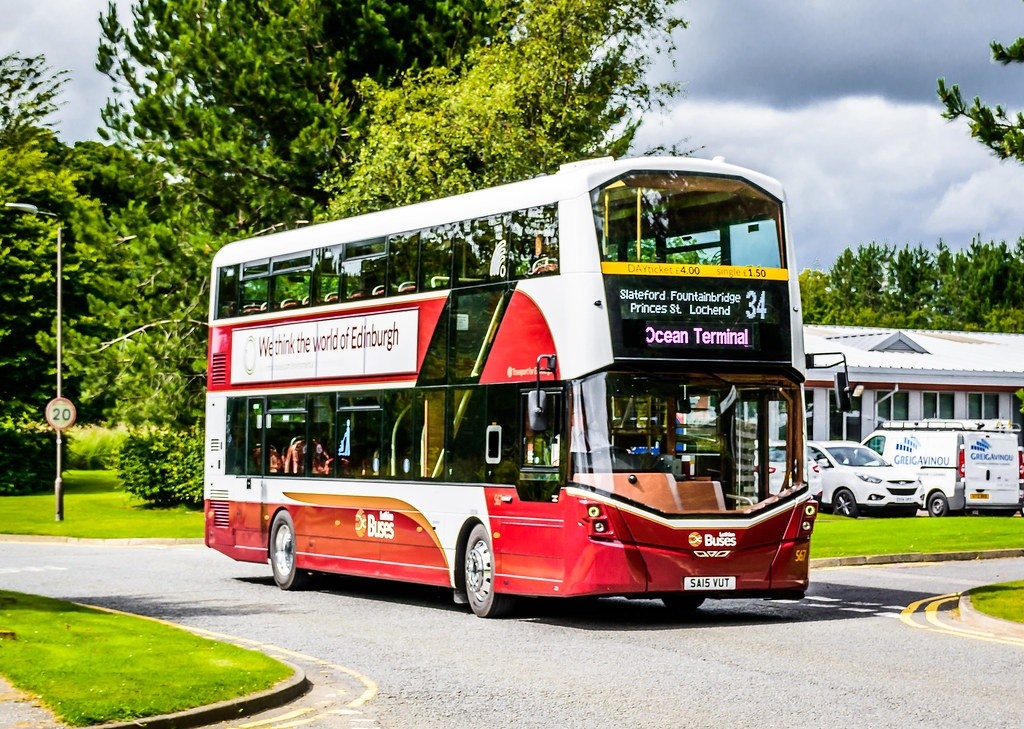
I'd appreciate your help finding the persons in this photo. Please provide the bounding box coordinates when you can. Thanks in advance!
[550,395,633,479]
[254,436,334,474]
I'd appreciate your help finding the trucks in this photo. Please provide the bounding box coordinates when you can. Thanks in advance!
[852,418,1022,518]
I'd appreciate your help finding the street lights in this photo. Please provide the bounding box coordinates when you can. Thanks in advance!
[1,200,63,519]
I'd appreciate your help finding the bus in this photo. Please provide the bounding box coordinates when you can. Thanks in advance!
[203,154,855,618]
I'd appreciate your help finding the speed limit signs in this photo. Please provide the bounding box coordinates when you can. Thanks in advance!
[46,397,76,430]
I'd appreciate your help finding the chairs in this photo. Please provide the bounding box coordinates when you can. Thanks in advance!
[252,434,374,476]
[531,257,559,275]
[243,280,416,316]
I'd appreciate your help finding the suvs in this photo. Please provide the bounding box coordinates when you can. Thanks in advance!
[754,439,925,519]
